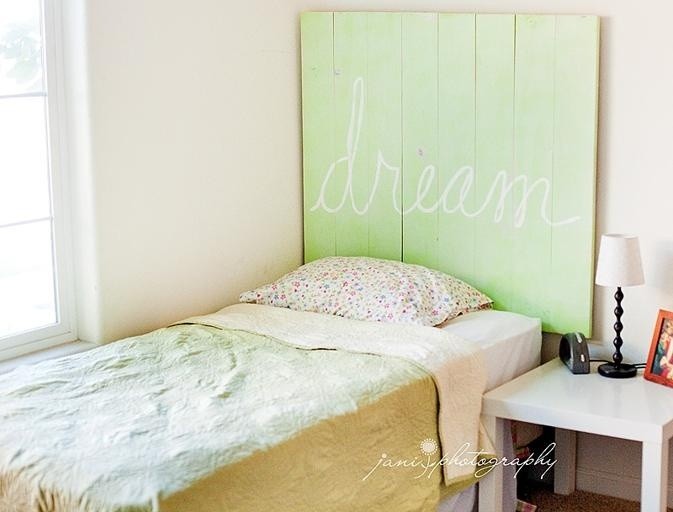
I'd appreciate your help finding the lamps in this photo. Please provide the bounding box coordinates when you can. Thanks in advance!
[595,233,644,378]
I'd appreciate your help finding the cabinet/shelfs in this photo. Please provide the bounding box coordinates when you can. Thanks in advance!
[479,355,673,512]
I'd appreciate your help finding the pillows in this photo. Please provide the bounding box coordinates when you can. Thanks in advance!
[238,256,493,332]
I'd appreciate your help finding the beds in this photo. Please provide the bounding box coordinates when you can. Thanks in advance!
[0,300,543,512]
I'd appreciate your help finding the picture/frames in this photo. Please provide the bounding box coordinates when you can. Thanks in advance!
[644,308,673,388]
